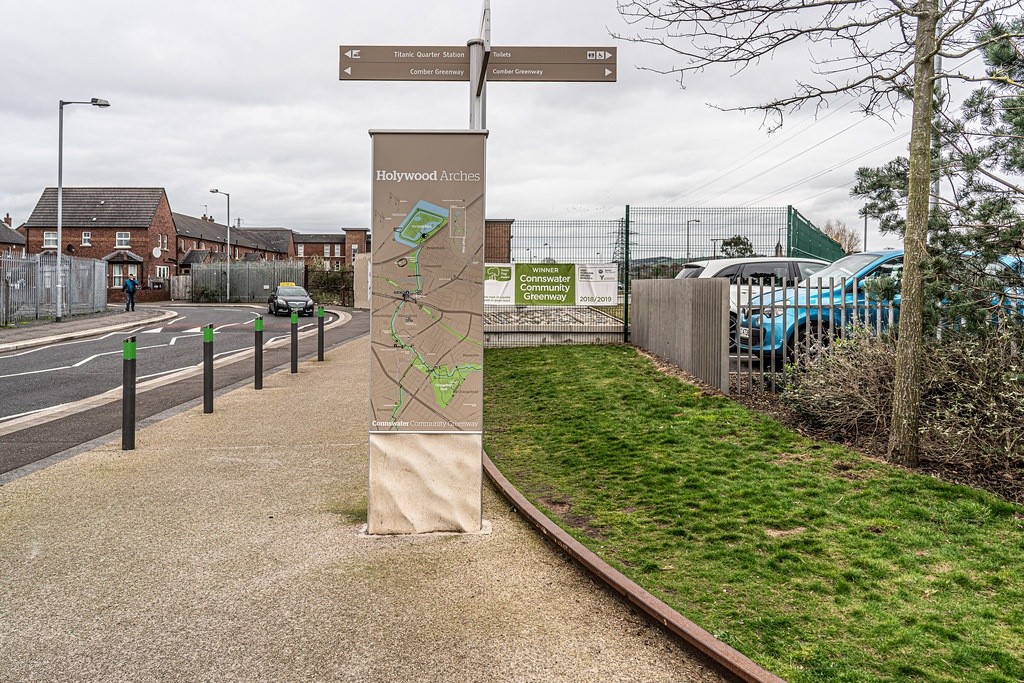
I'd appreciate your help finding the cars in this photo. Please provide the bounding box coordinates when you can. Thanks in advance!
[267,282,315,317]
[674,256,854,352]
[734,250,1024,374]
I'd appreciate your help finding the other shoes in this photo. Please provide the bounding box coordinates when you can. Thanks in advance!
[132,308,134,311]
[126,307,129,311]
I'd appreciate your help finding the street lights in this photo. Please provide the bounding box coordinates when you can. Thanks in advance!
[686,219,701,262]
[777,226,788,255]
[526,247,533,265]
[56,98,110,323]
[710,238,719,258]
[210,188,231,304]
[596,252,604,263]
[543,242,552,261]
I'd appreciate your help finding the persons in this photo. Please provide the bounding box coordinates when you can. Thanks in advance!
[122,274,138,312]
